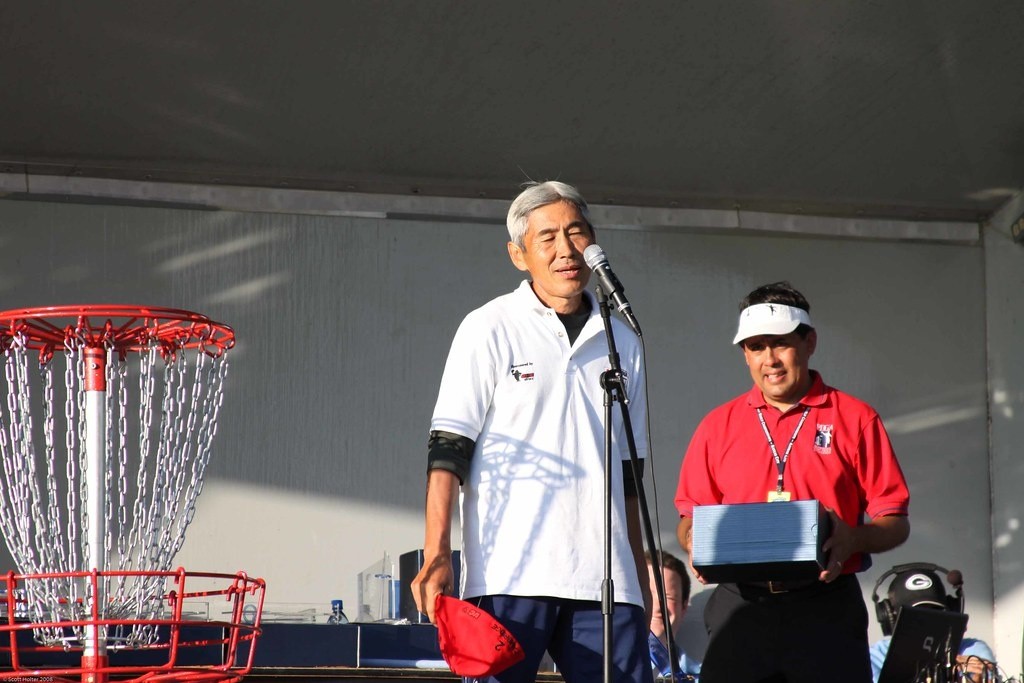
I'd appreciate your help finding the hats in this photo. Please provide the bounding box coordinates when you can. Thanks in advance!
[887,568,947,615]
[435,596,526,678]
[732,303,815,346]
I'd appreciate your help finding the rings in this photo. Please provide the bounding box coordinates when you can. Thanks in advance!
[837,561,841,566]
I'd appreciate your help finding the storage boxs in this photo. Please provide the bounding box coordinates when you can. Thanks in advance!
[691,499,832,586]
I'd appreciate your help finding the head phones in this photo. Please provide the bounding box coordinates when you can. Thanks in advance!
[872,562,965,637]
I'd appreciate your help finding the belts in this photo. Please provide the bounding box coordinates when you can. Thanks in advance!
[723,581,819,599]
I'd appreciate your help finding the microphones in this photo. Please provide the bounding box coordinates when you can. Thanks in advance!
[583,243,643,337]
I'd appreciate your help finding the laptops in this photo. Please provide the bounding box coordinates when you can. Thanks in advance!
[878,606,969,683]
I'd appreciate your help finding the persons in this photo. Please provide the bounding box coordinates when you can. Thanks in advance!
[673,282,910,683]
[642,549,700,683]
[411,182,648,683]
[868,569,997,683]
